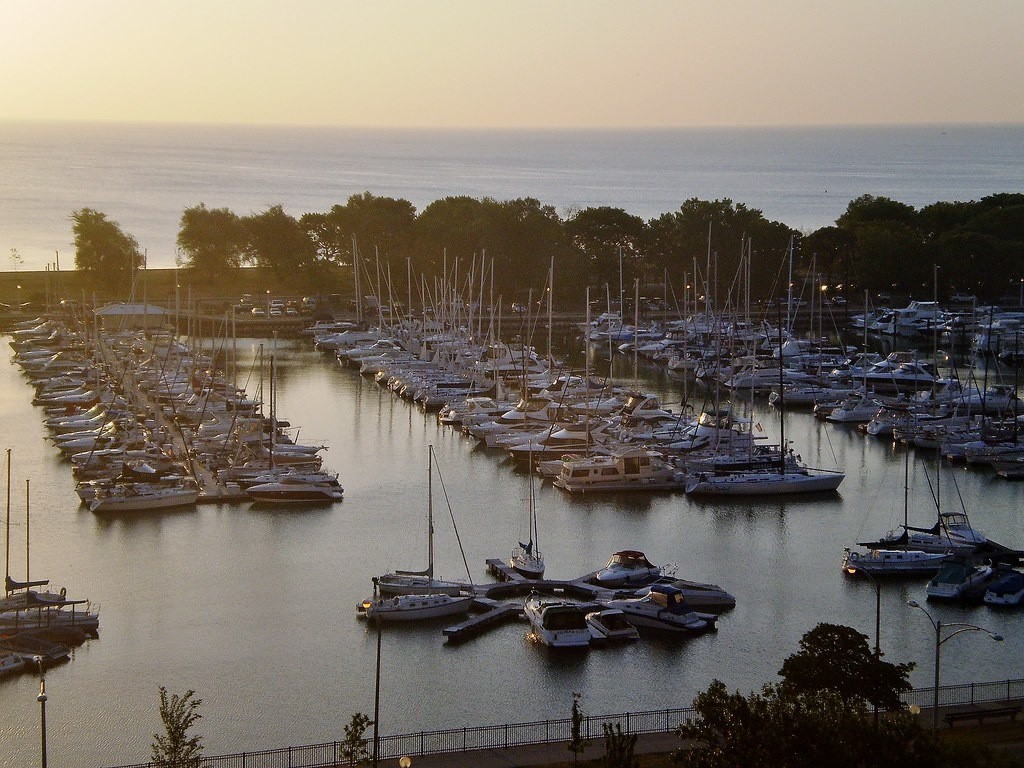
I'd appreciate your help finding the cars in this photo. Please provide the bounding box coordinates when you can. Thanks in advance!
[773,298,789,308]
[251,307,265,317]
[817,298,832,307]
[104,301,124,307]
[60,300,88,309]
[646,304,659,311]
[285,308,298,316]
[287,301,298,307]
[833,296,847,306]
[422,307,433,314]
[381,306,389,313]
[271,300,283,307]
[18,302,50,312]
[300,307,312,316]
[270,308,281,316]
[515,305,526,313]
[0,302,11,312]
[792,298,807,309]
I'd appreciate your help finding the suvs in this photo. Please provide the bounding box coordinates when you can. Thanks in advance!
[952,294,976,304]
[348,300,357,313]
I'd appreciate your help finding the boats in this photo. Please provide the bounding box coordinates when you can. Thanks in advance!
[372,572,464,596]
[855,512,987,556]
[925,562,993,598]
[524,600,591,647]
[585,550,736,642]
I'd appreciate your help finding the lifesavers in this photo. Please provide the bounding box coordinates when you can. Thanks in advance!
[60,588,67,596]
[594,458,605,461]
[851,552,859,561]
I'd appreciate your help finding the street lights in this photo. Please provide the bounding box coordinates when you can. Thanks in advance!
[266,289,270,320]
[908,599,1004,738]
[845,565,1003,745]
[363,599,381,768]
[32,655,49,768]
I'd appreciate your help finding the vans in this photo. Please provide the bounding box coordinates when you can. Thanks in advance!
[392,301,406,316]
[300,297,316,309]
[449,299,464,310]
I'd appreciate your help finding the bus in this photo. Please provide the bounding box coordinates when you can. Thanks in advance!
[364,296,378,314]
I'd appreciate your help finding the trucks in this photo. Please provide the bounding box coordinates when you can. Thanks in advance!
[878,291,890,302]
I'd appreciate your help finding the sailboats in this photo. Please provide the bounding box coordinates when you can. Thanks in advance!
[510,439,544,579]
[317,237,377,347]
[0,449,100,676]
[303,240,356,333]
[867,263,1024,479]
[842,422,947,576]
[826,288,980,421]
[347,246,848,494]
[367,445,477,621]
[9,249,344,512]
